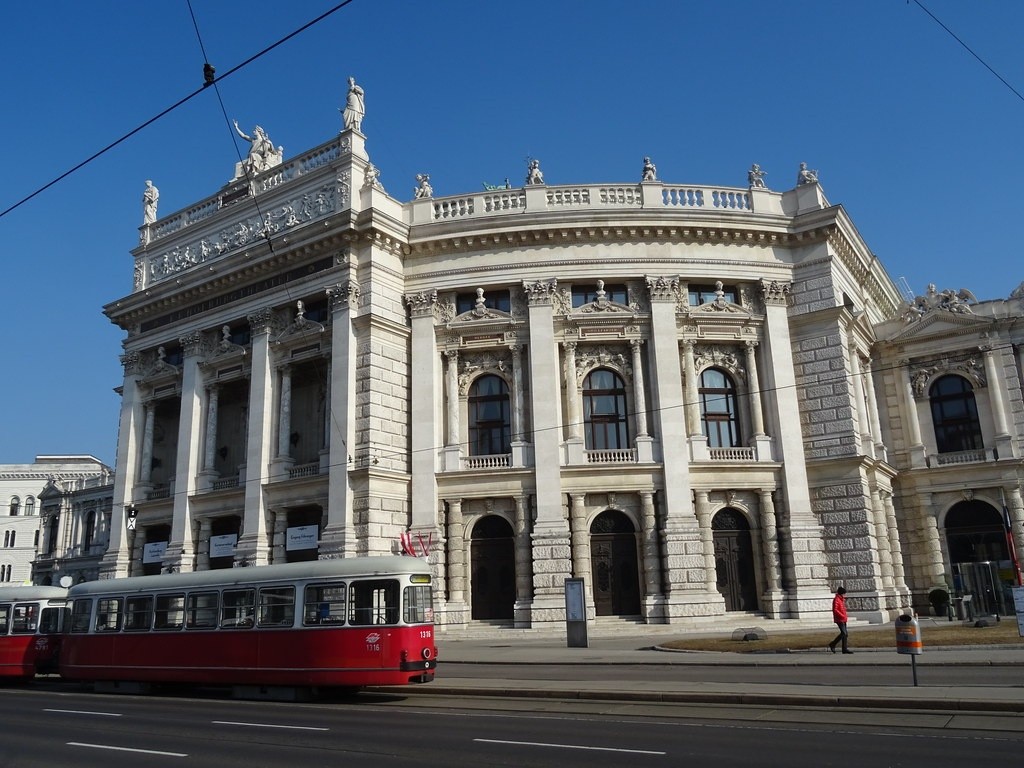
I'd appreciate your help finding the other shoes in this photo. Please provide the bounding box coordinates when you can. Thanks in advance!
[829,641,835,653]
[842,649,853,654]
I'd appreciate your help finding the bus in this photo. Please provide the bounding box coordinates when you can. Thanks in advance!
[57,554,438,693]
[0,585,68,679]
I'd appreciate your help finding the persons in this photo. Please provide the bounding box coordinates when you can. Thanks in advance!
[903,283,975,327]
[797,162,818,184]
[142,179,159,224]
[748,164,768,188]
[340,76,365,132]
[642,156,656,181]
[232,119,274,179]
[527,159,544,185]
[829,587,853,654]
[415,174,433,198]
